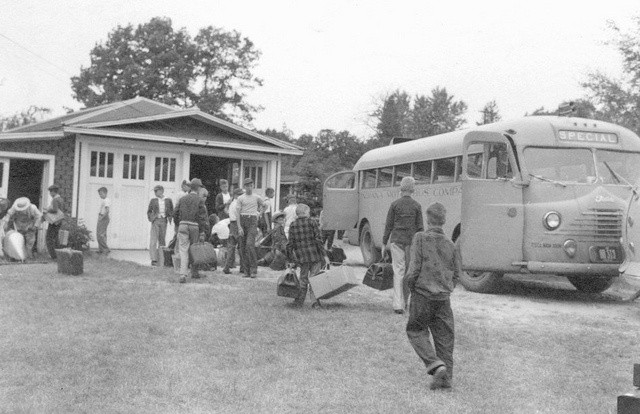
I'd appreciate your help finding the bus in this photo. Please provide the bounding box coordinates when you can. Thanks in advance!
[317,116,640,295]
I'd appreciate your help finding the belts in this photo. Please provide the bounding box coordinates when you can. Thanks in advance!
[99,214,103,216]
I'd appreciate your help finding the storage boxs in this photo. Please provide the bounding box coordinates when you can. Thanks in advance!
[308,264,358,300]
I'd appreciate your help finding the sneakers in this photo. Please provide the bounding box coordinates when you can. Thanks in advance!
[430,366,446,390]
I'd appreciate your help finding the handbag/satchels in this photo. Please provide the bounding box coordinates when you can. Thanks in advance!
[277,268,300,298]
[159,238,177,267]
[44,205,65,225]
[189,243,217,266]
[362,258,393,290]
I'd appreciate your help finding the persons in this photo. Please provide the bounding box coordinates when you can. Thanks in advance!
[381,176,424,313]
[181,179,191,193]
[236,178,266,278]
[173,178,206,283]
[223,188,244,275]
[146,185,173,266]
[215,179,234,222]
[260,187,275,238]
[209,215,232,241]
[0,196,42,259]
[271,212,287,248]
[42,185,65,260]
[95,187,111,256]
[286,203,331,310]
[319,210,336,249]
[283,194,298,240]
[406,202,462,390]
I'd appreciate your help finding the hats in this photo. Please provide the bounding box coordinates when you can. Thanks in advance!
[244,179,254,184]
[272,213,286,221]
[14,197,30,211]
[152,185,163,190]
[186,179,204,188]
